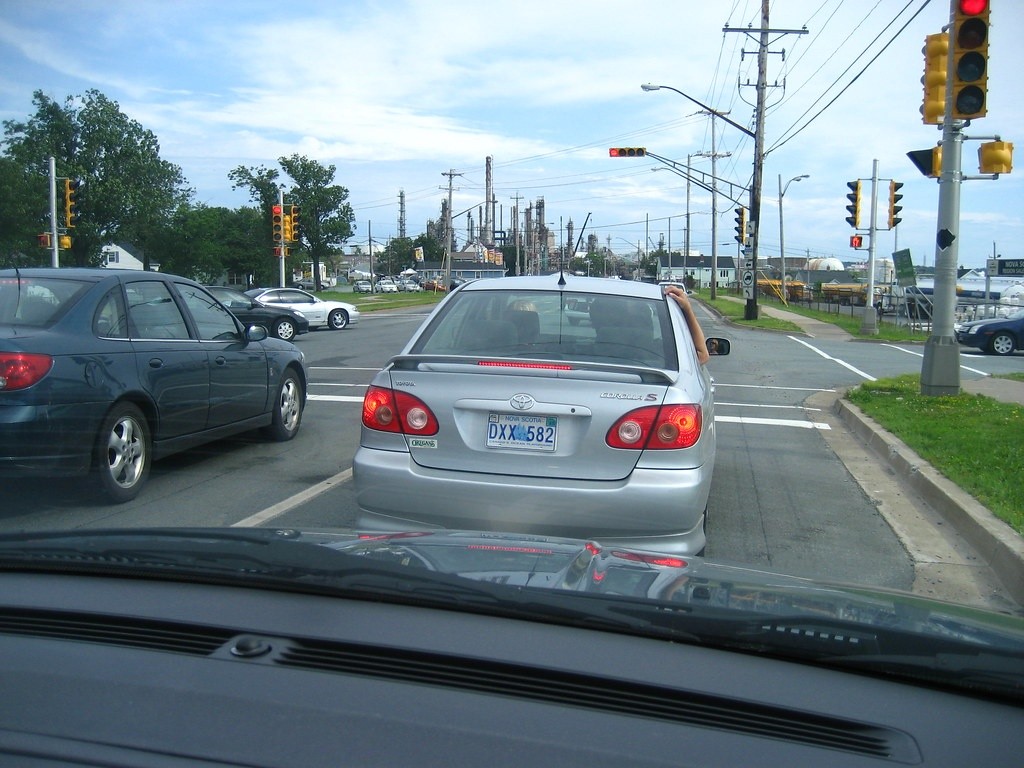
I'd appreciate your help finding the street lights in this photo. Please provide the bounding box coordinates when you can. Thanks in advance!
[650,167,716,301]
[640,83,764,321]
[445,200,498,294]
[779,173,810,304]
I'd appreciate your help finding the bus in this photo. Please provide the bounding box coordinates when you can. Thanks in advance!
[823,282,889,307]
[756,279,814,304]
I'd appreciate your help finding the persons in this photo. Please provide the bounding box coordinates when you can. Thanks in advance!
[708,338,720,354]
[664,284,709,366]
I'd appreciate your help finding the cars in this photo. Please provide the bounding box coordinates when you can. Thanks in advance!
[352,281,372,294]
[375,280,398,293]
[409,275,468,292]
[221,287,360,332]
[0,268,308,504]
[957,309,1024,356]
[295,278,330,290]
[564,296,595,325]
[352,276,732,562]
[397,280,420,293]
[658,281,692,296]
[201,285,309,342]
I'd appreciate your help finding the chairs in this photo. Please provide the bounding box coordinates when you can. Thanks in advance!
[592,326,656,361]
[507,310,540,349]
[455,322,521,351]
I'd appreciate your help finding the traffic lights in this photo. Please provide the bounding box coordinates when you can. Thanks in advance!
[906,146,942,177]
[273,247,280,256]
[845,180,862,228]
[290,205,303,242]
[38,235,50,247]
[734,207,746,245]
[951,0,989,119]
[272,206,282,241]
[609,146,646,158]
[284,247,293,257]
[61,236,75,249]
[850,236,862,248]
[65,179,83,229]
[888,181,904,230]
